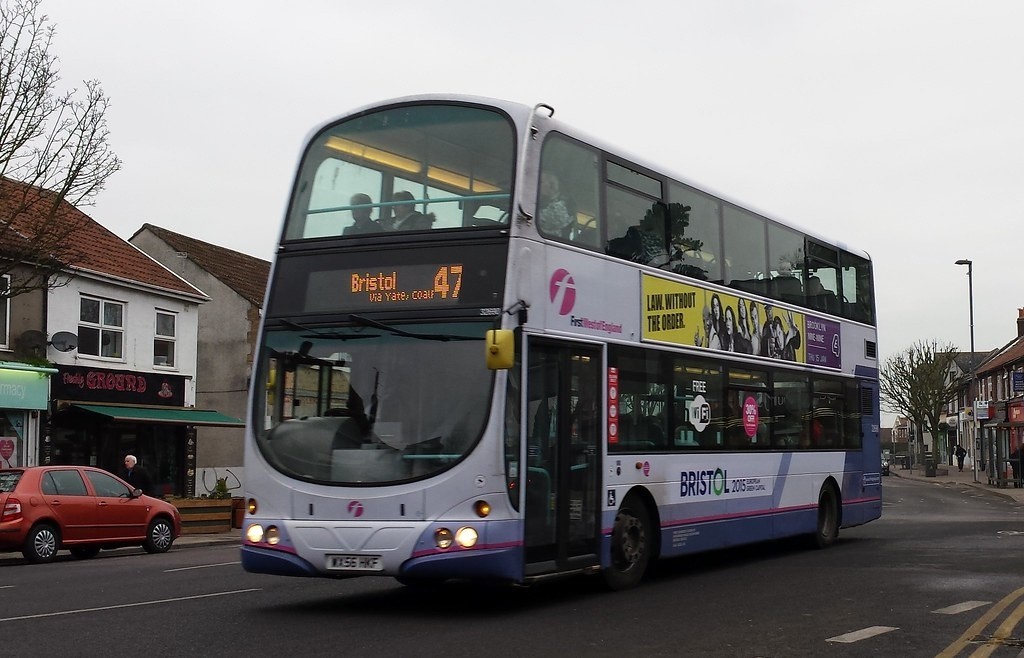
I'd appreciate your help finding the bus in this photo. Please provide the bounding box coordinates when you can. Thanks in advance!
[239,92,884,592]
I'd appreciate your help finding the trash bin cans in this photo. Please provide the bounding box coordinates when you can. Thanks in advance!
[926,459,937,477]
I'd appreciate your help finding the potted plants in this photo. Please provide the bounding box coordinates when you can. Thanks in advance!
[160,477,233,536]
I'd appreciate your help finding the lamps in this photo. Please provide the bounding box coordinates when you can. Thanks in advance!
[46,331,79,352]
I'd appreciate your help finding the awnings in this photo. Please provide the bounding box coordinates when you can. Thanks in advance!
[74,404,246,429]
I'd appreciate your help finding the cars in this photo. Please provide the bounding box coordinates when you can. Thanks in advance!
[880,452,889,476]
[0,465,184,563]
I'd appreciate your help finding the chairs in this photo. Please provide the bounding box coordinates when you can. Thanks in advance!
[576,217,851,317]
[58,475,81,494]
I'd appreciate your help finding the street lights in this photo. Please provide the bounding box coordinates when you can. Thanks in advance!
[955,259,976,484]
[908,435,915,474]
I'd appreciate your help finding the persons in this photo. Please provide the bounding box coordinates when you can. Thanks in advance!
[776,262,803,303]
[382,190,436,230]
[955,445,967,472]
[693,293,801,362]
[808,275,839,312]
[344,193,383,233]
[124,455,149,495]
